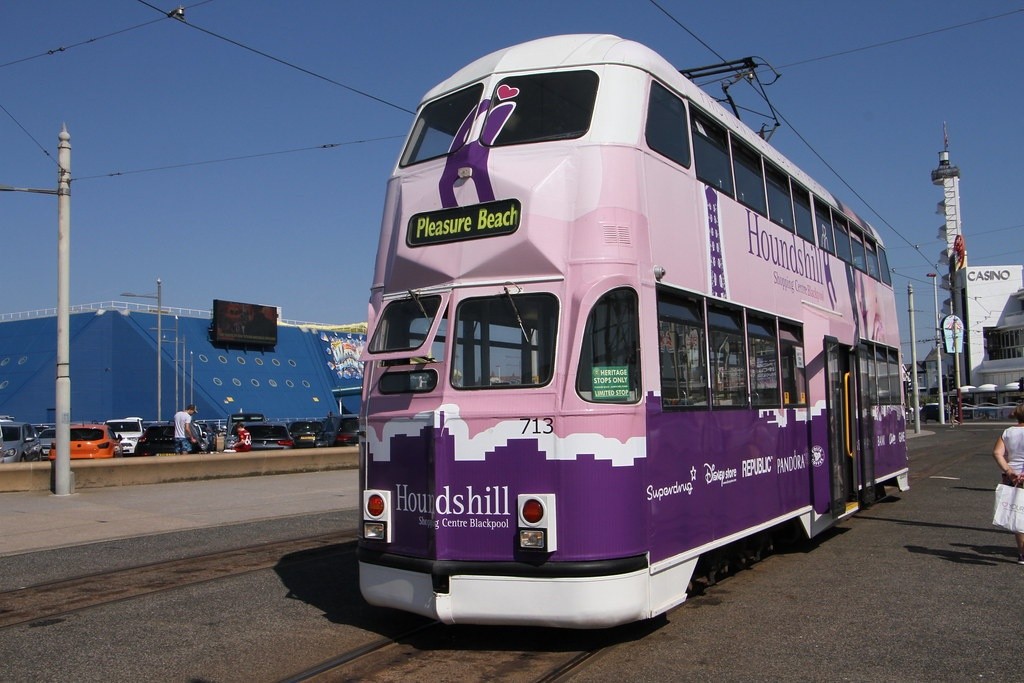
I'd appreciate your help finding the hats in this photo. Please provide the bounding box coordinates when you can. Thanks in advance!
[188,405,198,413]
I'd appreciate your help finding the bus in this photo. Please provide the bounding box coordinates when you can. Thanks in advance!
[356,29,913,632]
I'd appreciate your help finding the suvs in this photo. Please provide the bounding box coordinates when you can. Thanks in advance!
[314,414,360,448]
[0,421,44,464]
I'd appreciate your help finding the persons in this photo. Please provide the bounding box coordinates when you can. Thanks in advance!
[174,405,198,455]
[950,405,960,429]
[992,404,1024,564]
[232,421,252,452]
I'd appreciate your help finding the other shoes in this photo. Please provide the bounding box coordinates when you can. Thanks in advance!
[1017,555,1024,564]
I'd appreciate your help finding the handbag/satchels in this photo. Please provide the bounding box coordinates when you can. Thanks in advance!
[992,480,1024,535]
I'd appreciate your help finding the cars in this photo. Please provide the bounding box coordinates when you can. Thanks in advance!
[905,401,952,423]
[47,423,123,461]
[30,416,323,462]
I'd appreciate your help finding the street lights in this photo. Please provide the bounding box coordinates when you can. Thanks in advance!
[161,336,185,412]
[0,119,71,498]
[172,351,194,406]
[926,273,946,424]
[149,314,179,414]
[119,277,162,423]
